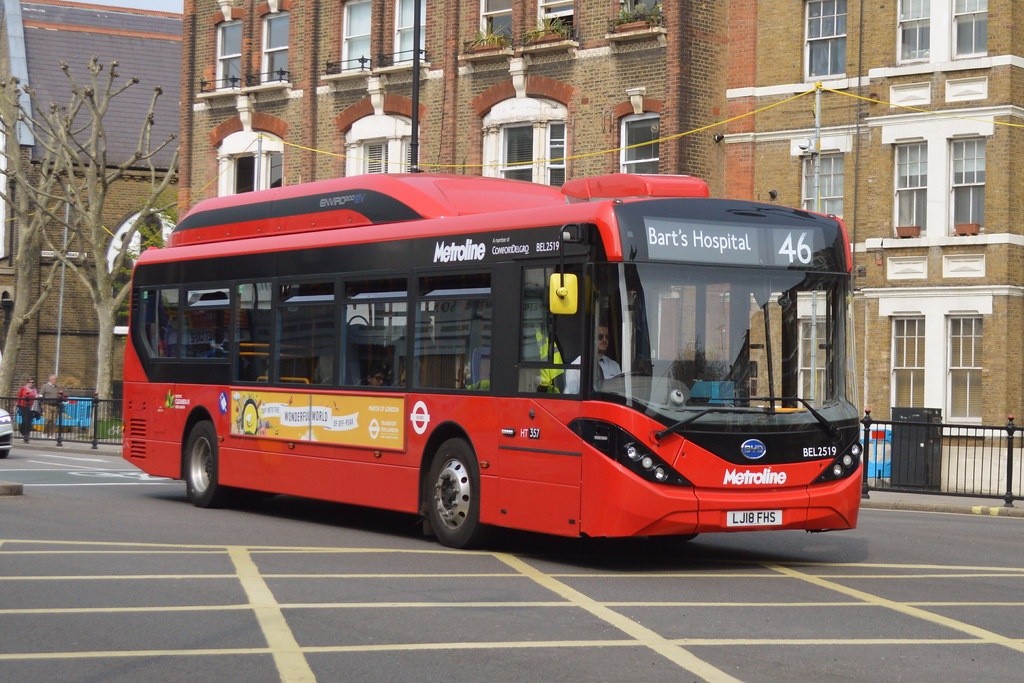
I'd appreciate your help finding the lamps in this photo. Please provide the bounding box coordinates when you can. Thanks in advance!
[714,133,724,142]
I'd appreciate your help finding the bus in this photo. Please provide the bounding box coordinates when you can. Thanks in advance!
[119,172,865,540]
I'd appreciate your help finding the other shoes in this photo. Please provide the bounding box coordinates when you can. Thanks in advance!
[42,433,47,438]
[24,435,29,441]
[49,435,55,439]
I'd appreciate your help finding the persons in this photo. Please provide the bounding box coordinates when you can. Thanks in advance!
[564,319,623,395]
[13,374,62,444]
[368,367,386,385]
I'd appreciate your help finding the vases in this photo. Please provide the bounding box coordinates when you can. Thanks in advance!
[897,226,920,238]
[955,223,980,235]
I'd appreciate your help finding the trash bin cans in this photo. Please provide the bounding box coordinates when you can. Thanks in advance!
[890,407,943,492]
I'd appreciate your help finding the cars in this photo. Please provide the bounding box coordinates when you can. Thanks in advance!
[1,408,14,457]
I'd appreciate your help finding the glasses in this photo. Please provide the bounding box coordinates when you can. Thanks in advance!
[28,382,35,384]
[598,334,609,341]
[372,376,385,383]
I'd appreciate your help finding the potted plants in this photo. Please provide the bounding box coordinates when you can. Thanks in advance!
[615,3,661,32]
[469,25,511,51]
[525,15,573,45]
